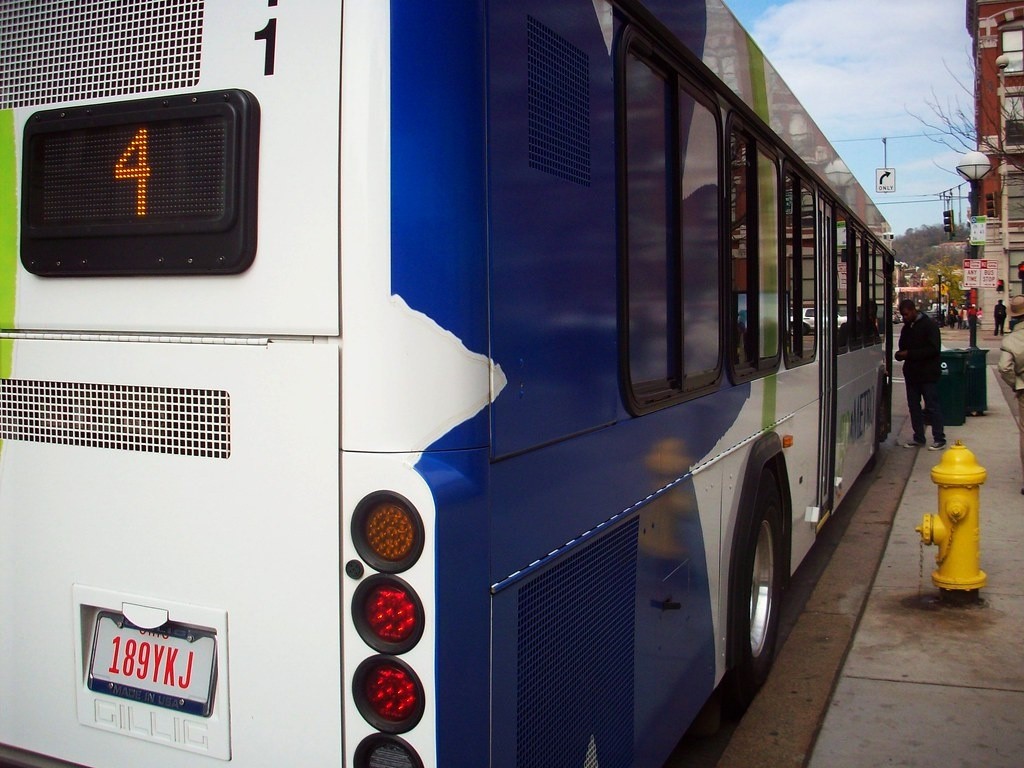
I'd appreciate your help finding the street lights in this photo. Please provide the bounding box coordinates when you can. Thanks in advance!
[937,272,943,325]
[954,150,994,347]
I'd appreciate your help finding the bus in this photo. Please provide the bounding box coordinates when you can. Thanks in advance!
[892,287,949,324]
[0,0,900,768]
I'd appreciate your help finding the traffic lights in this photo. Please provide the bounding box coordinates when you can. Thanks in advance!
[943,210,951,232]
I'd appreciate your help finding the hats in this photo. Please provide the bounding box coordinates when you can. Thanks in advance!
[1005,294,1024,317]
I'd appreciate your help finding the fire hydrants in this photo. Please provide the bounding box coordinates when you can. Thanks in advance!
[915,439,987,603]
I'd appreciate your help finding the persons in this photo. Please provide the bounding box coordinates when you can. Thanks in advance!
[940,302,983,330]
[994,300,1006,336]
[839,299,882,361]
[998,293,1024,495]
[895,299,947,451]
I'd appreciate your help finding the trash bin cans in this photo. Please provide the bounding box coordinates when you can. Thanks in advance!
[964,345,990,417]
[940,348,970,428]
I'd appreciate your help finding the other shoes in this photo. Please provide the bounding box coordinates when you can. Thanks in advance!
[903,438,926,448]
[928,438,947,452]
